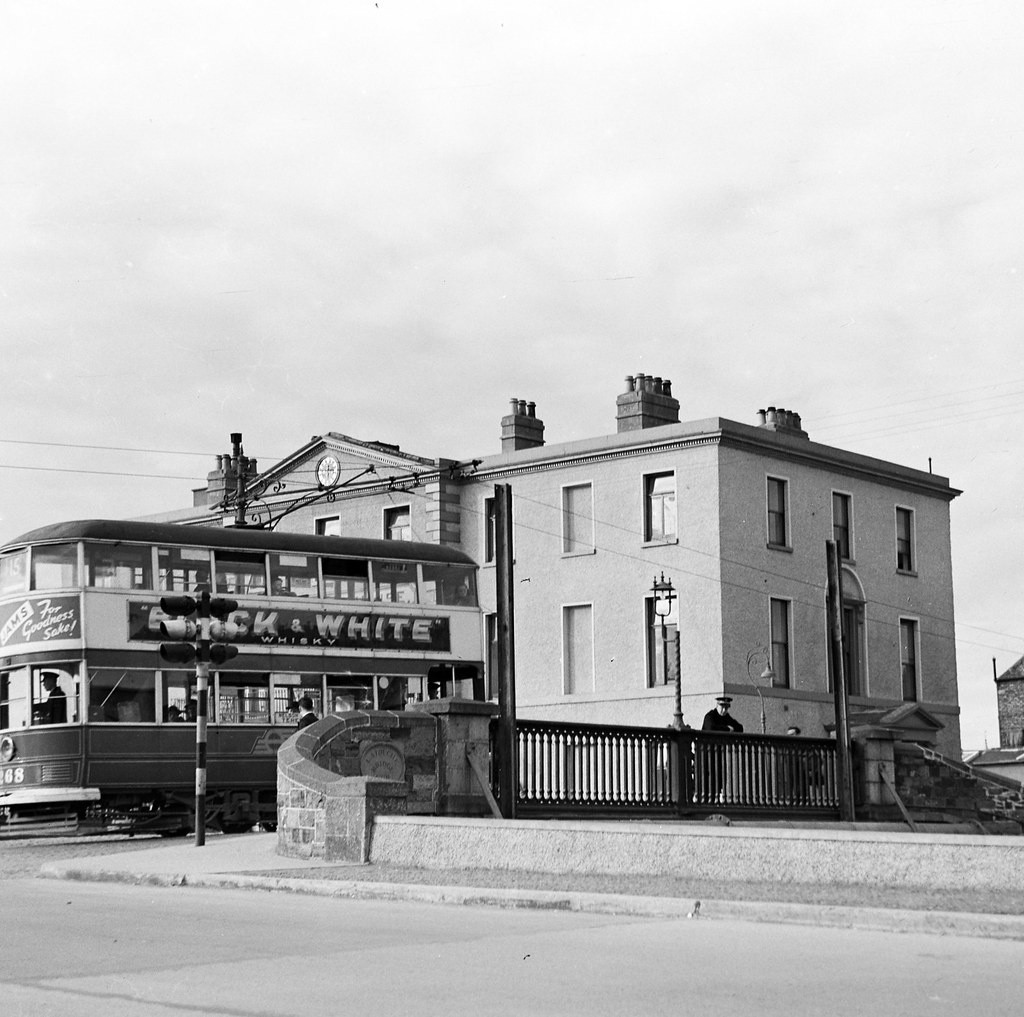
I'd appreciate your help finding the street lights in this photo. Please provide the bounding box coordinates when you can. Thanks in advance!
[160,596,197,662]
[208,600,241,666]
[746,643,775,735]
[651,573,688,731]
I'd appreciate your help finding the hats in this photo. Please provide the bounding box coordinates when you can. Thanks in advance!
[169,706,181,714]
[716,697,732,707]
[184,700,196,707]
[286,701,299,709]
[40,672,59,681]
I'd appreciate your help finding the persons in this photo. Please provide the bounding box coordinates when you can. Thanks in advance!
[285,701,300,713]
[192,569,211,592]
[271,578,285,596]
[32,671,67,726]
[297,693,319,731]
[183,699,197,723]
[452,583,475,608]
[787,727,801,736]
[701,697,744,803]
[215,571,229,593]
[168,705,186,723]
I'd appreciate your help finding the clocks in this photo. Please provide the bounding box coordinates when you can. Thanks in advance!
[315,454,341,486]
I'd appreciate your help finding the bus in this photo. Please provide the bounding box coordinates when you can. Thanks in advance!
[1,520,490,834]
